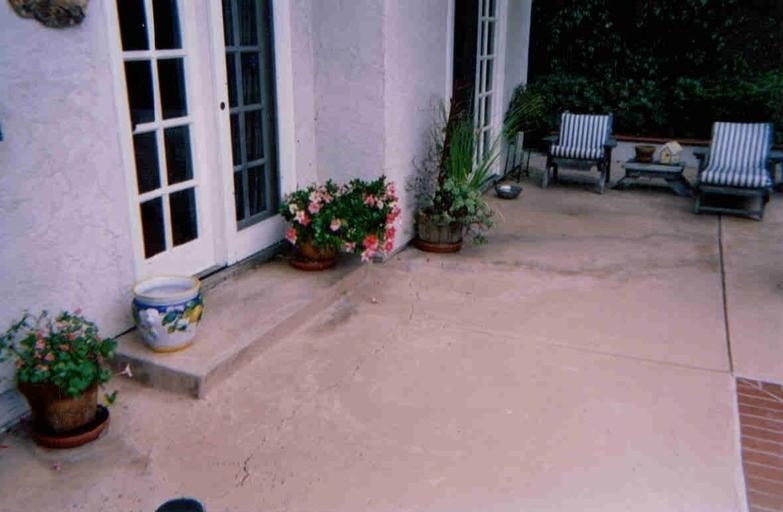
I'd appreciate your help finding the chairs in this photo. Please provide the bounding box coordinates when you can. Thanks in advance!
[692,121,782,222]
[539,108,617,195]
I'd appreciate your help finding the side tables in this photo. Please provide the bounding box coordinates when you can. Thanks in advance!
[610,159,694,199]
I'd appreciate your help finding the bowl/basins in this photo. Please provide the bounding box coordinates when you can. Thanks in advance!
[495,185,522,199]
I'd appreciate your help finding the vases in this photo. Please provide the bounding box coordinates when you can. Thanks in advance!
[285,223,348,272]
[635,144,655,163]
[130,275,204,352]
[18,380,112,450]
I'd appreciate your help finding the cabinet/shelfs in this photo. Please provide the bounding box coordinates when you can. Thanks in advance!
[502,130,534,184]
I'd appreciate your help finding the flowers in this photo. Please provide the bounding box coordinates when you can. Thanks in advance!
[278,173,403,264]
[0,305,133,404]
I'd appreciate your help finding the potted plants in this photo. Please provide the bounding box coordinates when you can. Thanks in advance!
[403,93,537,252]
